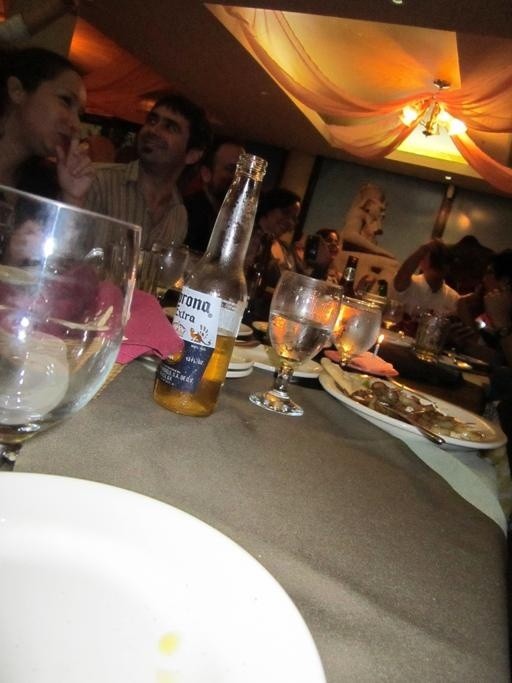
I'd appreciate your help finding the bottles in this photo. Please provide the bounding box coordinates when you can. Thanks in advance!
[338,255,360,296]
[379,281,389,297]
[243,233,276,320]
[154,151,268,418]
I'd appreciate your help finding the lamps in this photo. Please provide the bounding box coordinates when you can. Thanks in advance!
[400,79,467,137]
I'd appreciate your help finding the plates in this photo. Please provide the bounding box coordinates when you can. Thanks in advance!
[438,354,473,371]
[378,328,412,349]
[229,346,255,369]
[323,348,399,379]
[163,305,254,338]
[252,320,333,349]
[0,470,327,683]
[251,341,323,378]
[317,370,507,450]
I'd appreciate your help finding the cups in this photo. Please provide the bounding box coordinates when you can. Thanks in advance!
[414,311,451,363]
[111,244,162,295]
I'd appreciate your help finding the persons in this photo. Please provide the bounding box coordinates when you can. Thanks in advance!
[179,140,248,255]
[241,187,303,291]
[59,90,212,277]
[393,234,511,440]
[294,233,331,281]
[314,227,343,286]
[0,45,98,275]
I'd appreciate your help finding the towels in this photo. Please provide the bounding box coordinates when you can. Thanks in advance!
[0,282,185,365]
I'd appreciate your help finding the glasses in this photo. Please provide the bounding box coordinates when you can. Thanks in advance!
[279,206,300,225]
[483,267,494,280]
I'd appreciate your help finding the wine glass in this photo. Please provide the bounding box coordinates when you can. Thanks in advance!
[361,291,389,314]
[382,298,407,330]
[151,241,189,302]
[0,183,142,472]
[249,270,345,418]
